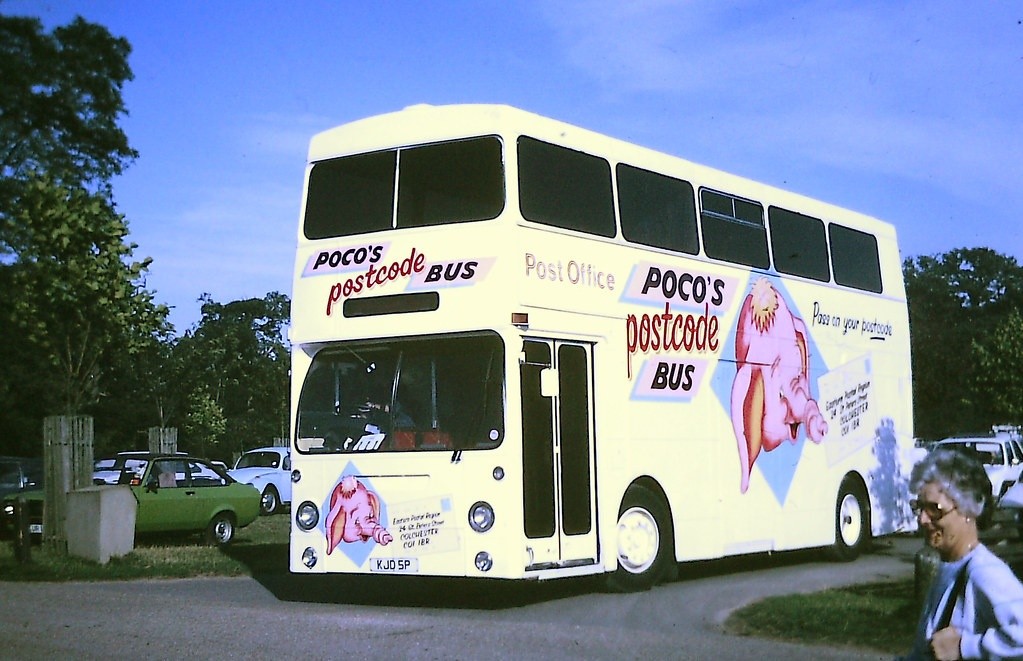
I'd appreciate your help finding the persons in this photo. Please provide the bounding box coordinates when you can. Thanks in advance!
[908,450,1022,661]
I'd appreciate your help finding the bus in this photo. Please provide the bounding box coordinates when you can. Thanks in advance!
[287,103,925,594]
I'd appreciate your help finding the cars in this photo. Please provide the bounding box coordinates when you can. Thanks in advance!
[227,446,292,517]
[931,435,1023,504]
[2,452,262,547]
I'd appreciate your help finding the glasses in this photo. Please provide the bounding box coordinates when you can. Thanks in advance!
[908,498,955,520]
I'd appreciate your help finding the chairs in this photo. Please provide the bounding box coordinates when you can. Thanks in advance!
[118,421,501,498]
